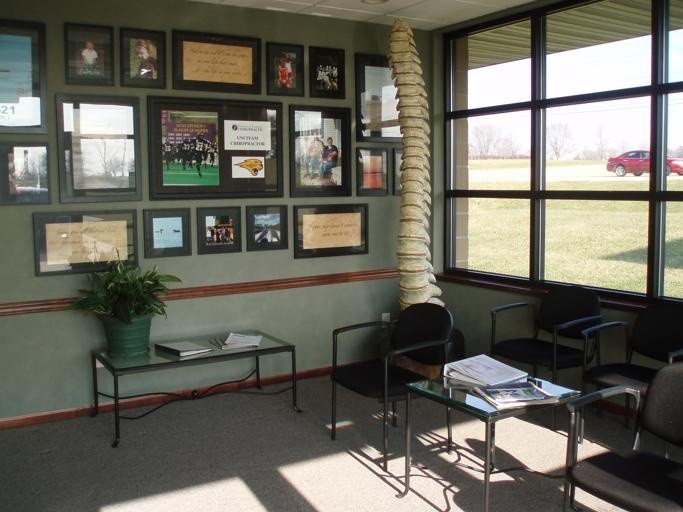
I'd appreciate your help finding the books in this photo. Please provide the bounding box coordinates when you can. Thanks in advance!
[473,381,560,410]
[443,376,528,390]
[208,331,262,350]
[154,341,214,357]
[443,353,528,387]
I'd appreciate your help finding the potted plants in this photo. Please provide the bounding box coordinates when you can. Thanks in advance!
[69,245,183,360]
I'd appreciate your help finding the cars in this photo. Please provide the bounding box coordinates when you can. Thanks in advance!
[602,149,683,177]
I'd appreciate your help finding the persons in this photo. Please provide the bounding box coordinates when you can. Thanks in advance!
[282,53,293,84]
[317,64,338,90]
[134,41,158,81]
[278,61,289,88]
[79,41,101,80]
[163,133,218,179]
[300,129,324,182]
[317,137,338,181]
[207,226,230,243]
[324,151,337,161]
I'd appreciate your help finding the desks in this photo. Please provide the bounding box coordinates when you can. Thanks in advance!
[88,330,304,447]
